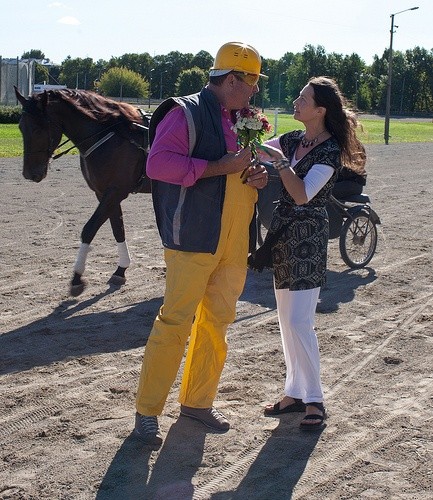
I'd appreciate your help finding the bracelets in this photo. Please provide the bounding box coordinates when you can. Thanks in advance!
[273,157,291,172]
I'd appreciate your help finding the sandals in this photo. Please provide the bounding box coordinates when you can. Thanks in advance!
[265,396,308,414]
[300,402,326,431]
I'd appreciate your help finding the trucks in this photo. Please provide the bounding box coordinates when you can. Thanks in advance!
[34,84,67,95]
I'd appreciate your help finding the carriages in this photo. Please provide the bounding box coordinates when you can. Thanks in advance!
[12,81,381,288]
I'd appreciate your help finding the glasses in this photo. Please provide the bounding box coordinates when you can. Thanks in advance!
[233,71,259,87]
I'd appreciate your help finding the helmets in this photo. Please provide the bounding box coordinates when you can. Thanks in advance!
[209,42,260,75]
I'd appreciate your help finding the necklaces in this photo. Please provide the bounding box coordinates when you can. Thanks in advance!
[301,130,327,149]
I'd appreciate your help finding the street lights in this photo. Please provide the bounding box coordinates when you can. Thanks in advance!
[384,7,420,145]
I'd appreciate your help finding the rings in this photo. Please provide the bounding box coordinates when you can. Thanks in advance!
[264,173,268,179]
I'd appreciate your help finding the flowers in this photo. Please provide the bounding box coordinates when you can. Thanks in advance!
[230,105,271,163]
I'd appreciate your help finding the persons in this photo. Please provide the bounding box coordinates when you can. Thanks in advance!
[134,42,268,445]
[243,75,367,430]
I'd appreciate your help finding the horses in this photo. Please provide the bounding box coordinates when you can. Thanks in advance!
[13,84,266,295]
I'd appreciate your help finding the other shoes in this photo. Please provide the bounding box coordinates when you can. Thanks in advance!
[133,412,163,446]
[179,403,231,431]
